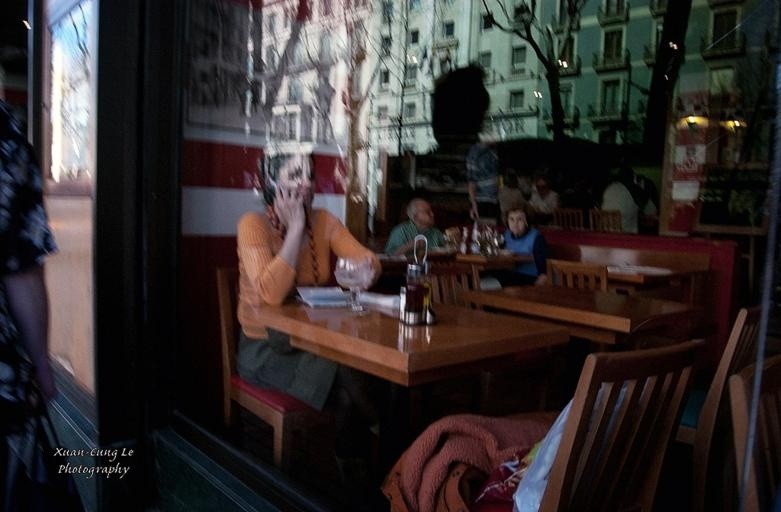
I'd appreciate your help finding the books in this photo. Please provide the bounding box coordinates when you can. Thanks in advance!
[291,284,351,310]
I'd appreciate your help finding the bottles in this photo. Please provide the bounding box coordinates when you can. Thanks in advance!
[459,219,500,256]
[398,263,433,327]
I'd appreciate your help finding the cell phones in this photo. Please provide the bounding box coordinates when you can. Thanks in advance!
[264,172,281,198]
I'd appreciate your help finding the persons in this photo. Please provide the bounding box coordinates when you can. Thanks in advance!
[382,195,463,259]
[227,136,399,493]
[497,173,526,221]
[466,122,502,224]
[465,207,550,315]
[602,164,658,237]
[528,175,560,222]
[0,98,60,510]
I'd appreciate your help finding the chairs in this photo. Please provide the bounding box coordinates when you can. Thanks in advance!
[216,208,781,512]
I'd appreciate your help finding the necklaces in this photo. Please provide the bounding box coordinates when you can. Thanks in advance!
[270,206,325,289]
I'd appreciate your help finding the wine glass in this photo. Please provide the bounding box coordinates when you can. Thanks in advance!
[333,254,377,318]
